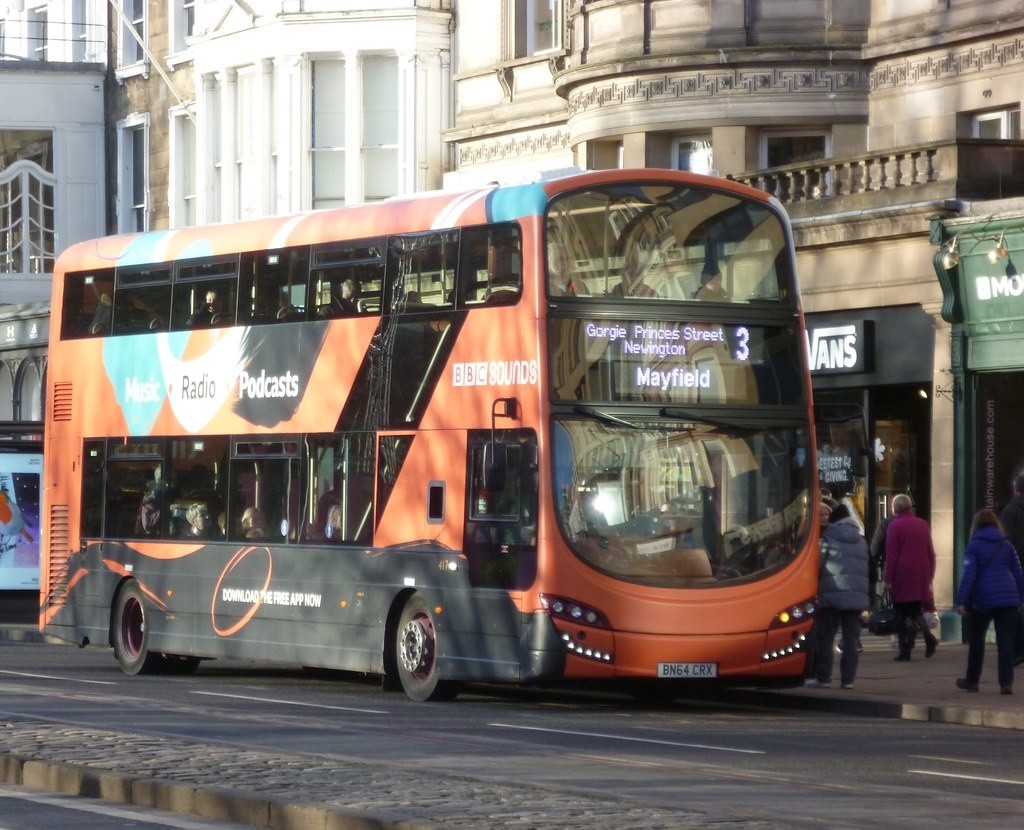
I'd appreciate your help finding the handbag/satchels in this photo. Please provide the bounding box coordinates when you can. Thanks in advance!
[867,587,899,635]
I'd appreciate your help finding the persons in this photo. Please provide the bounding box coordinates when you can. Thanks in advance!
[260,292,298,324]
[316,278,369,319]
[955,509,1023,694]
[547,241,591,298]
[87,274,142,330]
[131,462,178,539]
[869,515,920,649]
[821,487,864,653]
[689,259,730,301]
[237,506,269,542]
[177,501,218,540]
[610,261,659,297]
[1000,473,1024,570]
[308,489,344,541]
[805,503,868,687]
[403,290,422,303]
[184,287,230,328]
[884,494,938,662]
[819,502,834,538]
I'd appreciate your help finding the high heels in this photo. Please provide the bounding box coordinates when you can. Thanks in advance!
[924,635,939,657]
[895,648,911,662]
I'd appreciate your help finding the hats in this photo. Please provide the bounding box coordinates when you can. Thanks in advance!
[828,504,852,524]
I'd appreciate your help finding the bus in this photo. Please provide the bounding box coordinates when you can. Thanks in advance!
[39,168,821,700]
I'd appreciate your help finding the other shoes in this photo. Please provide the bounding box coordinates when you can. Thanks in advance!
[1000,685,1013,694]
[956,679,980,693]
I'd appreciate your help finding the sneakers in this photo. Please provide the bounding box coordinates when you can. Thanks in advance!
[807,678,831,688]
[840,682,854,689]
